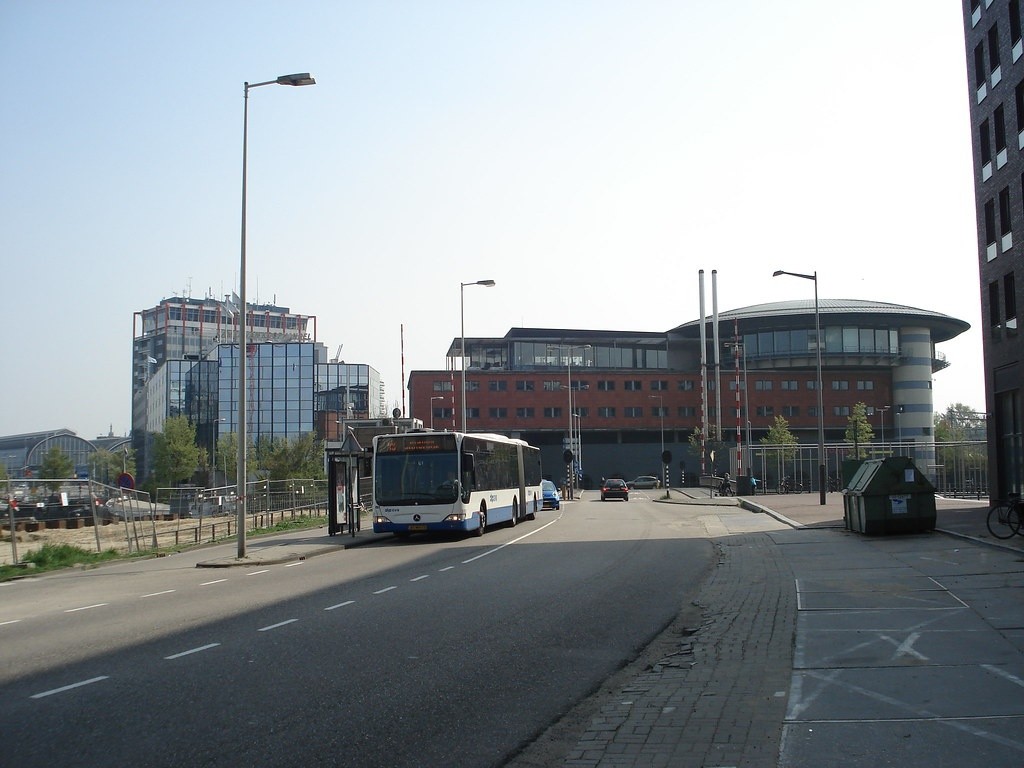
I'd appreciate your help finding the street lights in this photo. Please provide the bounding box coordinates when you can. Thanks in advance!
[572,412,583,488]
[773,269,829,506]
[567,341,591,436]
[210,418,227,488]
[875,405,890,459]
[969,453,978,495]
[459,279,497,434]
[723,340,752,477]
[430,396,444,429]
[648,394,668,489]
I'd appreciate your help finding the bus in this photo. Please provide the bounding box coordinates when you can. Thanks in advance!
[371,427,543,537]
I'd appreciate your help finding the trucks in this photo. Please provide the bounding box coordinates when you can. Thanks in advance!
[52,485,94,497]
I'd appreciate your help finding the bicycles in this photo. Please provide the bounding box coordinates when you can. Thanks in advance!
[986,492,1024,542]
[775,475,804,495]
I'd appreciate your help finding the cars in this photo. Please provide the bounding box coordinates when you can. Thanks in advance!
[625,475,661,490]
[601,479,628,502]
[542,478,559,510]
[2,484,52,504]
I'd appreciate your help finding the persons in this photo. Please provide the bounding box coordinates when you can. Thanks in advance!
[750,477,757,496]
[717,473,734,497]
[443,472,463,496]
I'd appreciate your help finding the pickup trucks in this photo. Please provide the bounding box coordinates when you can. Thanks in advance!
[191,495,236,516]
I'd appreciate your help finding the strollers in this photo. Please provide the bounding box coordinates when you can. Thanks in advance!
[713,481,728,496]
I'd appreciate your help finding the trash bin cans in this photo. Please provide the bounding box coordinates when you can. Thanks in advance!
[841,455,939,533]
[736,475,749,496]
